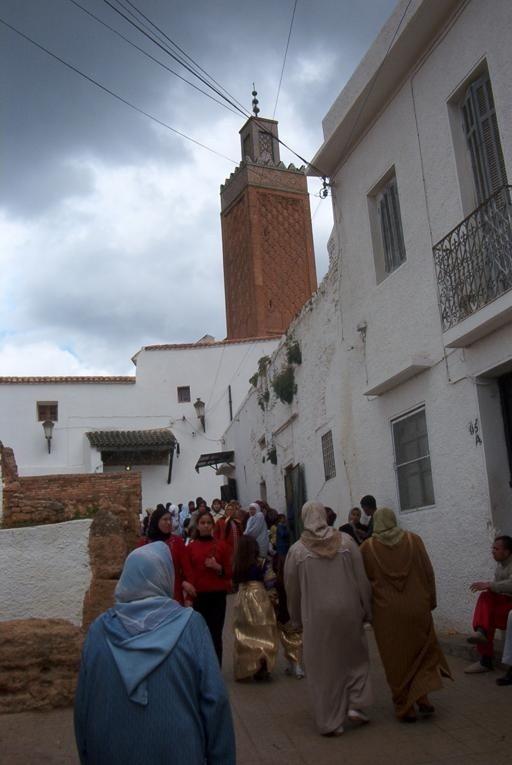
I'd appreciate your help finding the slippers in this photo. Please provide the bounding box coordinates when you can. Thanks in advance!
[466,631,488,645]
[465,661,490,673]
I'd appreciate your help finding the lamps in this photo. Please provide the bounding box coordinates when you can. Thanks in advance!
[193,397,206,434]
[42,418,55,453]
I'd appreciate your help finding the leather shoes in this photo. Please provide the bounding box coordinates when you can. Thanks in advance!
[334,725,345,735]
[347,709,370,723]
[400,708,417,724]
[417,695,436,713]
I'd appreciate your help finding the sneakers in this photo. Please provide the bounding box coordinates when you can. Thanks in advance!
[496,670,512,686]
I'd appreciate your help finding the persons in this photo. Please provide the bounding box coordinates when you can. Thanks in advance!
[462,536,512,686]
[284,501,372,734]
[359,508,455,723]
[74,540,236,765]
[138,496,379,681]
